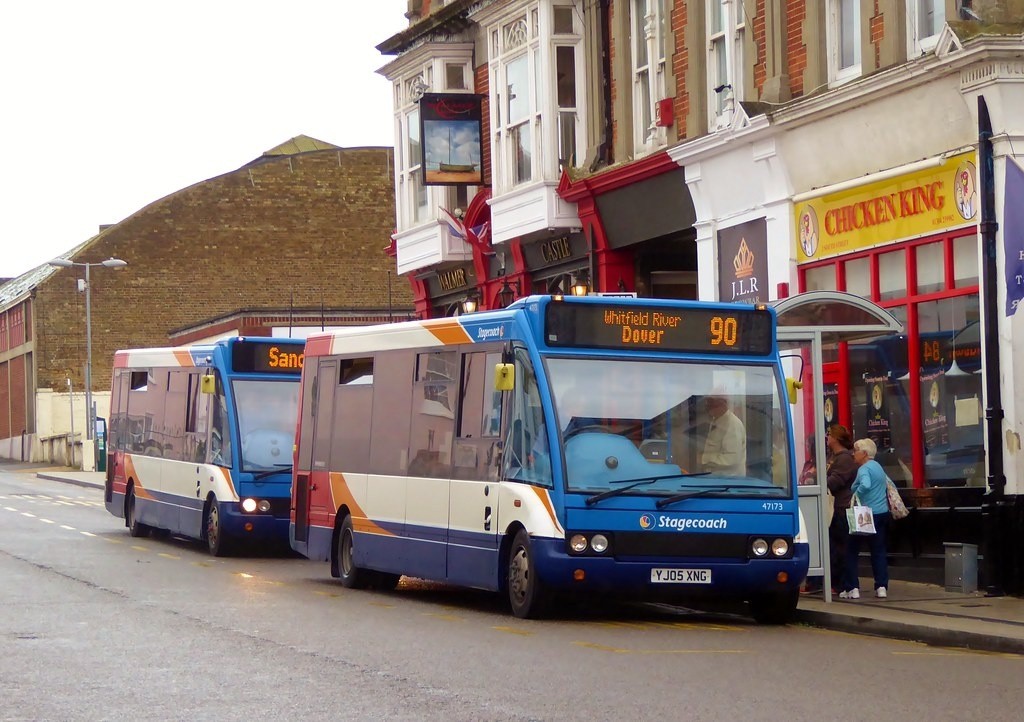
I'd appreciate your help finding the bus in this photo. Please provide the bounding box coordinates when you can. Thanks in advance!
[289,295,810,626]
[104,336,306,557]
[822,328,983,483]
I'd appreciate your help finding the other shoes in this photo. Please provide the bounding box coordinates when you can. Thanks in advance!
[799,584,823,594]
[830,586,839,595]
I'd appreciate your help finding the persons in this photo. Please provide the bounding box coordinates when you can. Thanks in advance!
[531,385,597,481]
[799,425,897,599]
[696,387,746,478]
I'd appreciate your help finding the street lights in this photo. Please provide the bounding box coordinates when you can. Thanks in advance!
[49,257,127,472]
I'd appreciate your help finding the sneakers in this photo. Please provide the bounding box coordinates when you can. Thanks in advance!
[839,587,860,599]
[875,587,888,598]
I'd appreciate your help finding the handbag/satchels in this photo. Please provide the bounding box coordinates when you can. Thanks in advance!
[826,488,835,527]
[886,478,909,519]
[846,493,876,536]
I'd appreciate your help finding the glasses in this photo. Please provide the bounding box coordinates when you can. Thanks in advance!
[826,433,832,436]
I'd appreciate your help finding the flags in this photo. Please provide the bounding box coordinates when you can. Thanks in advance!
[440,205,496,255]
